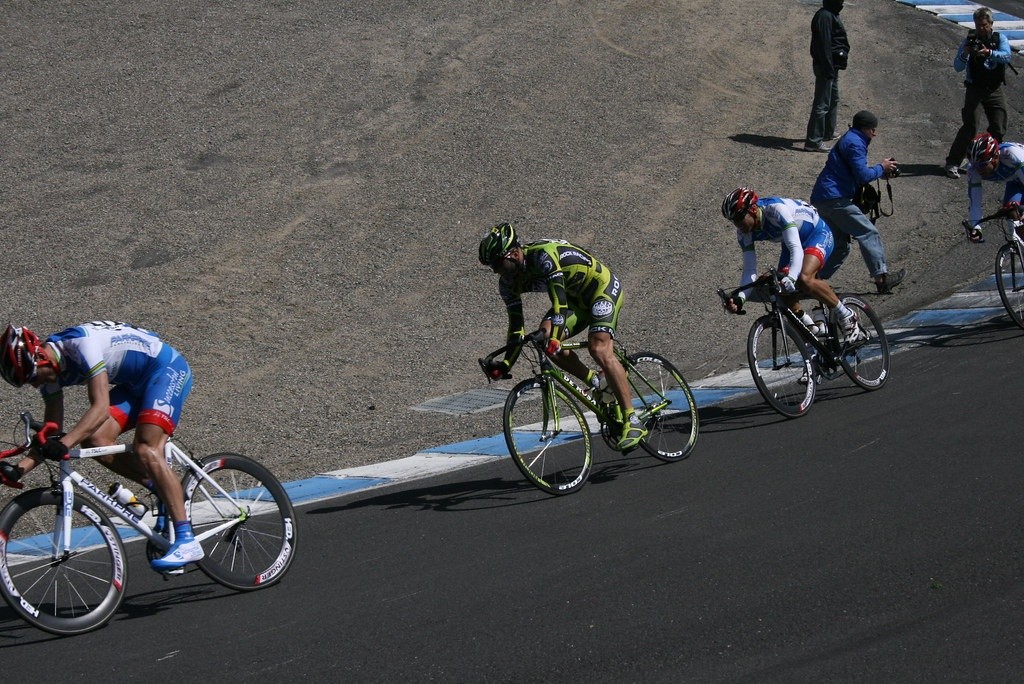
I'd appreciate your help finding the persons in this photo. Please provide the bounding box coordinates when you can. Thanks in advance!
[803,0,850,153]
[944,8,1011,179]
[810,111,905,296]
[0,320,205,569]
[966,132,1024,244]
[722,187,871,384]
[479,222,648,449]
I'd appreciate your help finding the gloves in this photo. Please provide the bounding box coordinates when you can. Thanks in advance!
[41,440,68,461]
[490,369,503,381]
[4,466,23,482]
[545,337,560,357]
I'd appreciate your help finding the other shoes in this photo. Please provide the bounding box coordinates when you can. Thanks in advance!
[804,142,831,152]
[823,132,842,140]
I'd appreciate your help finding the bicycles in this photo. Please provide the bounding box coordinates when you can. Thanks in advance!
[717,266,890,418]
[962,202,1024,328]
[479,326,699,495]
[0,411,297,633]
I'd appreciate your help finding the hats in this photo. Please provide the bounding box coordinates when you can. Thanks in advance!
[853,110,878,129]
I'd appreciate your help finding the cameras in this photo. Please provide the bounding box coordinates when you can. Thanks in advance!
[967,39,982,52]
[885,158,901,178]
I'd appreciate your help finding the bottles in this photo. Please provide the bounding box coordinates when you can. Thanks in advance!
[812,305,828,336]
[795,308,819,335]
[157,503,165,530]
[108,483,144,516]
[598,368,614,403]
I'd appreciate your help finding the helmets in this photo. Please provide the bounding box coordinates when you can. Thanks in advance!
[0,323,43,387]
[967,132,999,164]
[722,187,758,220]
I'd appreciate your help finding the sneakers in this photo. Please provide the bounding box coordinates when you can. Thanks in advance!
[152,503,166,532]
[798,365,822,385]
[618,407,647,451]
[839,306,860,345]
[150,524,204,570]
[585,369,604,423]
[876,269,907,293]
[944,164,960,179]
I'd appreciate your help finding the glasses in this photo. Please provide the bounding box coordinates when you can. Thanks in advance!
[25,364,38,384]
[478,222,518,265]
[970,158,993,169]
[491,249,513,267]
[730,208,747,221]
